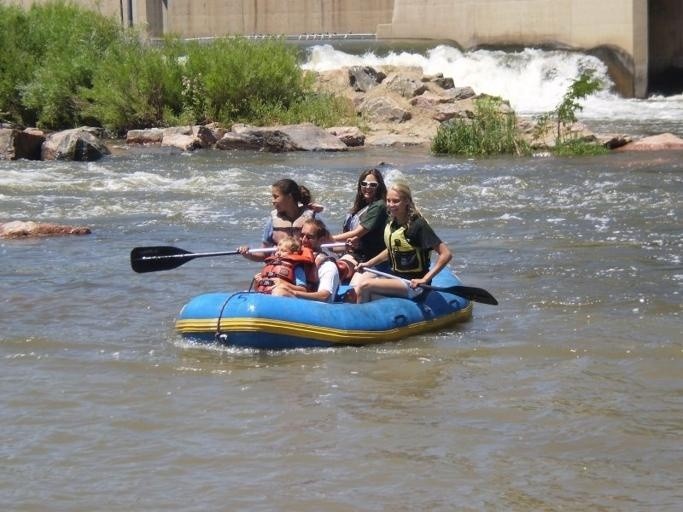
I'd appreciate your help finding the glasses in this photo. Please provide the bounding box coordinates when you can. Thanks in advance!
[358,181,378,188]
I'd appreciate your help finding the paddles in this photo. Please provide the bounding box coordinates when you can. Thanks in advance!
[131,243,348,273]
[362,269,496,304]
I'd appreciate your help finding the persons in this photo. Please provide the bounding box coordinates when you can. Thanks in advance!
[334,168,388,274]
[236,217,339,303]
[237,184,319,245]
[347,183,451,305]
[253,235,324,275]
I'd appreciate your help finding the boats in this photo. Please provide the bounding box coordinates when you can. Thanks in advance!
[171,257,476,357]
